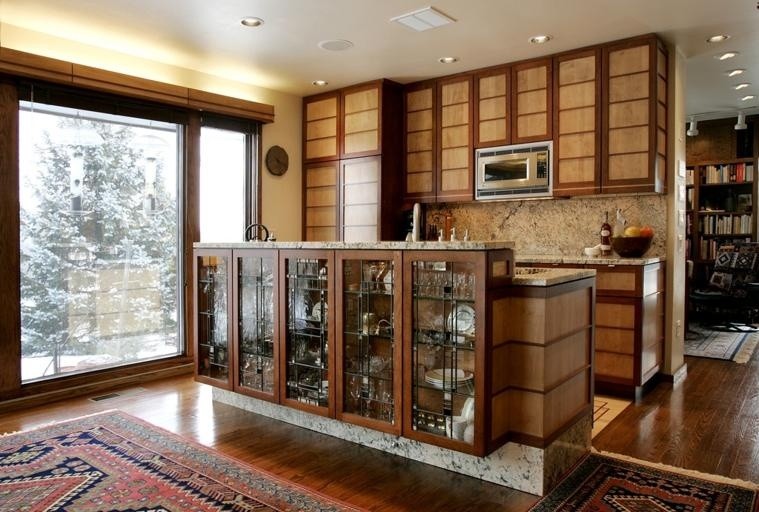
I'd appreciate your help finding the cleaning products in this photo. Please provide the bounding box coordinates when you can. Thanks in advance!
[463,229,471,241]
[438,229,445,241]
[450,227,458,241]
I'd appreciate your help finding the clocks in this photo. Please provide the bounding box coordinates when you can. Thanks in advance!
[266,145,288,175]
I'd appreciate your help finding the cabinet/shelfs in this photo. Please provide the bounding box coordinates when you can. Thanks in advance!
[686,158,758,323]
[233,249,335,419]
[336,250,486,459]
[193,248,232,391]
[515,262,667,402]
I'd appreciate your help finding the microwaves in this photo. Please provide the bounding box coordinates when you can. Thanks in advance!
[475,140,554,201]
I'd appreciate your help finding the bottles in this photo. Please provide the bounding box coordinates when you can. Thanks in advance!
[723,187,737,212]
[600,210,613,256]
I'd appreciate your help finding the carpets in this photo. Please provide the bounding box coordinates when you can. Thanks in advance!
[0,408,366,512]
[685,320,759,364]
[528,451,759,512]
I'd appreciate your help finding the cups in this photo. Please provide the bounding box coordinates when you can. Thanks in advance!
[446,416,467,441]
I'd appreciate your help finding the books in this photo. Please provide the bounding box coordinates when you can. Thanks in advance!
[684,162,755,186]
[687,188,695,211]
[685,237,751,261]
[686,215,753,234]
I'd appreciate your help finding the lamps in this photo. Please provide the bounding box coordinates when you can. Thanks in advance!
[733,111,748,130]
[686,119,698,136]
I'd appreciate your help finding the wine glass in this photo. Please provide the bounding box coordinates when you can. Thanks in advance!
[410,271,474,300]
[240,354,274,393]
[346,375,394,421]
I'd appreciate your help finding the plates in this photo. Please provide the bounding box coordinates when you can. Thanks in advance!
[425,303,475,388]
[461,395,475,445]
[311,301,328,321]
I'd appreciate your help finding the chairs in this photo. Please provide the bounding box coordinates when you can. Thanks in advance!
[689,240,758,323]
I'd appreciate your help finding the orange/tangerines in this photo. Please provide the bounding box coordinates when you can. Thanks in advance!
[613,232,621,239]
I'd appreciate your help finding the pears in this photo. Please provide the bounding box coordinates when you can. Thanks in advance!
[625,227,640,237]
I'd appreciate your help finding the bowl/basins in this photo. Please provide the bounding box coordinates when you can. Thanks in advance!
[584,247,600,256]
[607,234,653,259]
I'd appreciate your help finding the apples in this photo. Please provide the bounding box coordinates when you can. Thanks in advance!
[640,226,653,237]
